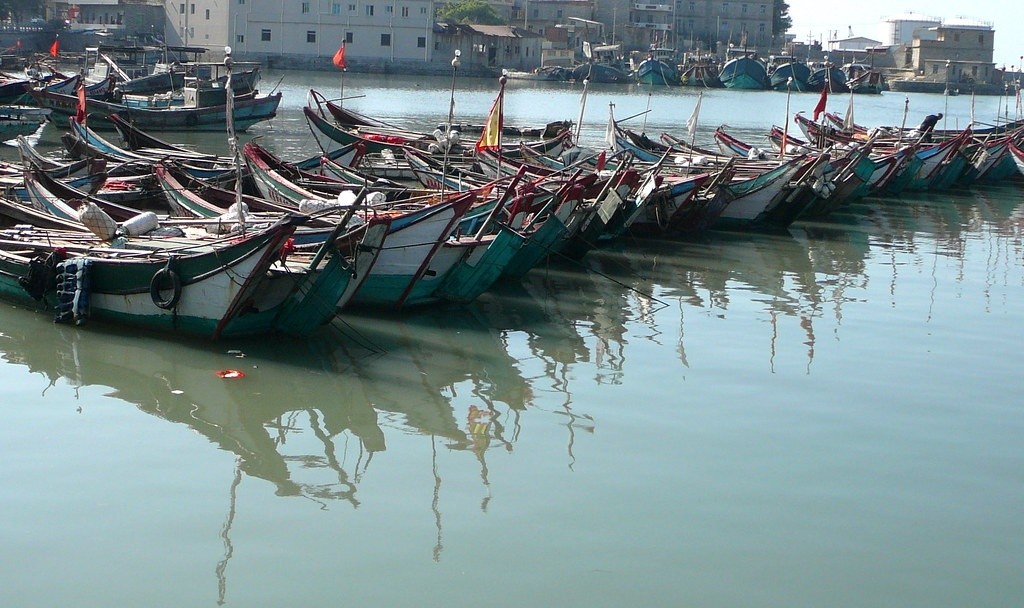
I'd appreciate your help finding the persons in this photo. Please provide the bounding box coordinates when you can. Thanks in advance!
[920,113,943,147]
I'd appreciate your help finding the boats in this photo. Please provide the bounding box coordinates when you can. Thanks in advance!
[503,25,887,95]
[944,87,960,95]
[1,35,280,137]
[0,89,1024,353]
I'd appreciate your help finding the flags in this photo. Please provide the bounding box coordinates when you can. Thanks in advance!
[687,95,701,136]
[606,109,618,152]
[76,81,87,126]
[50,40,60,58]
[814,85,827,122]
[843,94,853,131]
[334,46,346,68]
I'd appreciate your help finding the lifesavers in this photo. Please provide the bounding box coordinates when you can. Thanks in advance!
[149,269,182,310]
[187,113,198,126]
